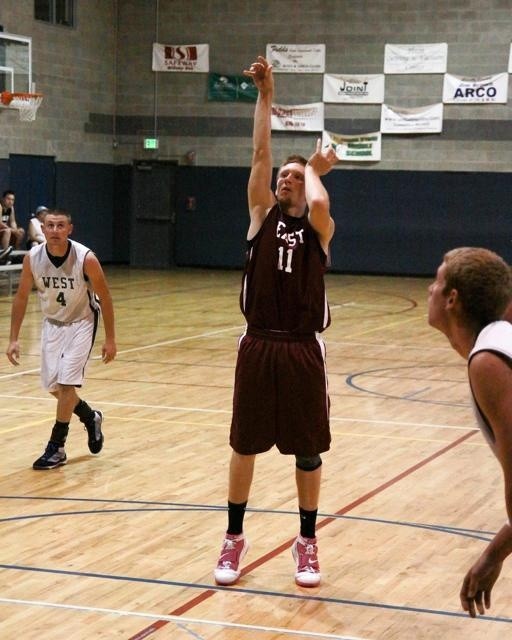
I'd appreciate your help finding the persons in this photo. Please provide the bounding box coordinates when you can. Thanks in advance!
[427,245,512,619]
[214,54,340,589]
[26,205,48,248]
[0,190,24,265]
[6,207,117,471]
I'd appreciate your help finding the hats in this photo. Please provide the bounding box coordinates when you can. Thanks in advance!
[35,205,49,215]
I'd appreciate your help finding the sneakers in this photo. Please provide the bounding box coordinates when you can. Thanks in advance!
[80,409,104,453]
[214,532,248,585]
[33,440,67,470]
[290,534,321,587]
[0,245,13,265]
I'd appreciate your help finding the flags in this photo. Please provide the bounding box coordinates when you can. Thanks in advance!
[269,100,325,133]
[265,42,325,74]
[206,71,259,103]
[442,72,509,105]
[321,130,382,162]
[382,41,448,74]
[322,73,386,105]
[379,102,445,134]
[150,41,209,74]
